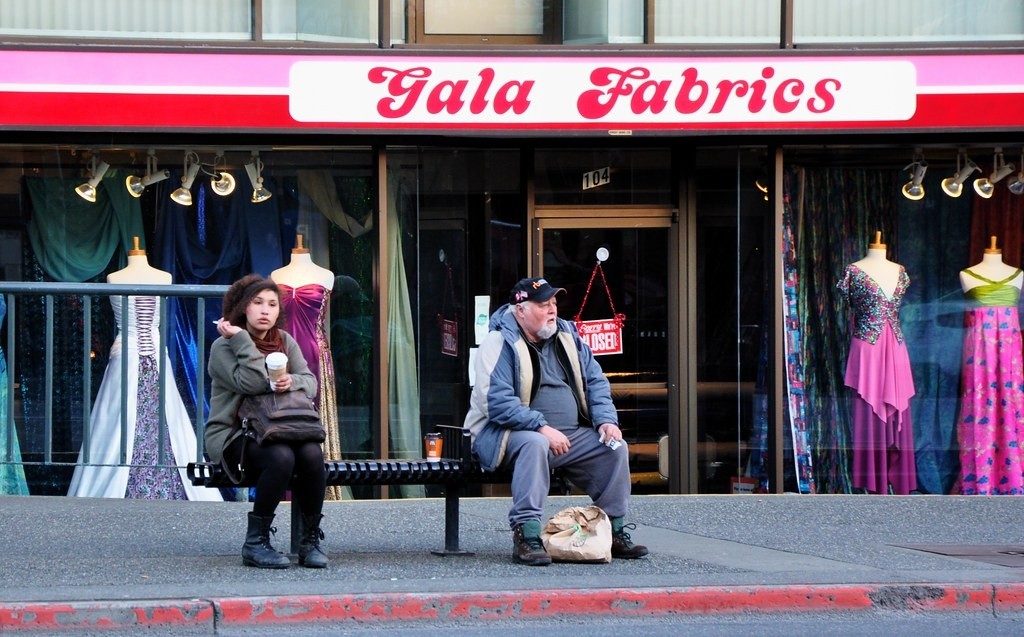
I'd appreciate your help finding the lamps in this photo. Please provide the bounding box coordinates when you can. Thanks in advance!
[1008,155,1024,195]
[903,148,928,200]
[243,150,272,203]
[973,146,1015,198]
[170,151,201,205]
[942,149,978,198]
[75,150,110,202]
[126,149,169,197]
[211,150,236,196]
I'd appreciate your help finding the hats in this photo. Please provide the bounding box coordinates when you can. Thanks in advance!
[510,276,564,304]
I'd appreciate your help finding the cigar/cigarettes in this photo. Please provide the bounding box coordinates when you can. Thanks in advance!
[213,320,224,325]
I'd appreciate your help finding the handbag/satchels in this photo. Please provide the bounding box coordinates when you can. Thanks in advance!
[236,389,327,444]
[540,505,612,564]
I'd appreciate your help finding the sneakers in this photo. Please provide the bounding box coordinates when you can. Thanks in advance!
[513,522,551,566]
[613,522,649,560]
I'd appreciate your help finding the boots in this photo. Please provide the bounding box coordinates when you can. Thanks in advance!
[298,512,329,569]
[241,512,291,569]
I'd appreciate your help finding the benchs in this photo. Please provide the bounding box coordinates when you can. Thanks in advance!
[188,425,568,556]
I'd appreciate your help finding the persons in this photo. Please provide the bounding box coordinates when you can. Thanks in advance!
[835,231,917,495]
[268,235,335,411]
[204,275,329,569]
[949,236,1024,495]
[462,276,648,566]
[64,238,225,502]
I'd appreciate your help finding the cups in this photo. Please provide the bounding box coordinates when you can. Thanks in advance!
[424,433,443,458]
[266,352,288,392]
